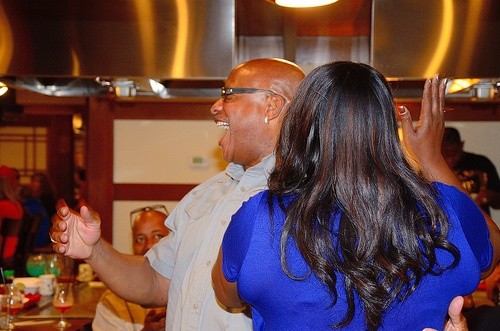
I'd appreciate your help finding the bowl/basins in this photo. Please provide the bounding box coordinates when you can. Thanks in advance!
[12,276,40,295]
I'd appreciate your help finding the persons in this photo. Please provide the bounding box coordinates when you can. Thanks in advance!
[211,61,500,331]
[53,59,467,330]
[442,127,500,216]
[91,204,169,331]
[0,165,55,267]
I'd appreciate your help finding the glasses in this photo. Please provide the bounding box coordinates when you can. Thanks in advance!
[220,87,275,101]
[130,205,169,228]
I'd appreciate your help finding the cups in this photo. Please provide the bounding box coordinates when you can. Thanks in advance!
[38,273,56,296]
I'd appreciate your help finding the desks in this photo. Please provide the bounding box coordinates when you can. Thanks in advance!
[12,285,106,331]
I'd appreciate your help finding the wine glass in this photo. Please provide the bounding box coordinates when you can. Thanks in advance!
[53,283,74,328]
[0,284,22,331]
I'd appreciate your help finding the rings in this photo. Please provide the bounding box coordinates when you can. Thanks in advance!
[49,232,57,243]
